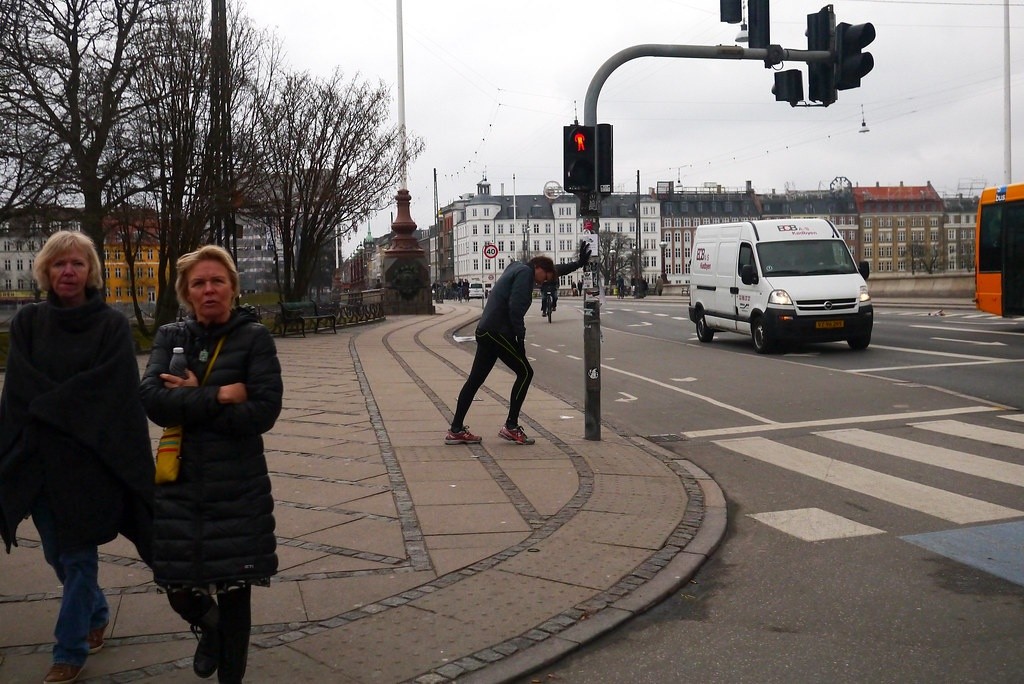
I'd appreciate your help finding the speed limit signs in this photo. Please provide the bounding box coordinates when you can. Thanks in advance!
[483,245,499,259]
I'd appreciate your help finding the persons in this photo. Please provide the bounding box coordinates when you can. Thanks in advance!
[444,241,592,445]
[656,276,663,296]
[631,276,649,297]
[616,276,624,299]
[541,277,559,317]
[135,245,284,684]
[0,231,156,684]
[432,278,469,302]
[572,282,576,294]
[578,280,582,295]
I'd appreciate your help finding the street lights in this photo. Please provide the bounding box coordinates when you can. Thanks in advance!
[657,240,668,284]
[609,249,617,285]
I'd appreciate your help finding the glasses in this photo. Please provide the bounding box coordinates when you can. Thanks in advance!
[544,272,548,283]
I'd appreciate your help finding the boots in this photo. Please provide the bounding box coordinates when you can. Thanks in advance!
[190,602,222,678]
[215,583,251,683]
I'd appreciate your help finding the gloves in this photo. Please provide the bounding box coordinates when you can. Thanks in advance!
[578,241,592,266]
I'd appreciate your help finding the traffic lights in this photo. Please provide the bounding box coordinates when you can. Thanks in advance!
[835,21,876,92]
[563,125,598,193]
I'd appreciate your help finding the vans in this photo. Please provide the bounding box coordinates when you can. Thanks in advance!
[688,219,874,354]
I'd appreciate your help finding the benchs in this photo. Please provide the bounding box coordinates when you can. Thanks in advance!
[277,299,337,338]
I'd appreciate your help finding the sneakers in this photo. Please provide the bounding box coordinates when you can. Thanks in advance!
[88,619,109,653]
[44,654,87,684]
[499,425,536,445]
[445,425,482,445]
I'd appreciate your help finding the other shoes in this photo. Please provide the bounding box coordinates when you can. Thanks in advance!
[542,311,547,317]
[552,306,556,311]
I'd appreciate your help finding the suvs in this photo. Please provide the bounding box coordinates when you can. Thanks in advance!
[468,283,483,299]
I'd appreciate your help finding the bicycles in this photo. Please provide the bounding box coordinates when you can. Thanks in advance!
[539,284,561,324]
[617,286,624,299]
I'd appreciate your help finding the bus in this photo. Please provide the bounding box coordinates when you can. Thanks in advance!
[971,183,1024,318]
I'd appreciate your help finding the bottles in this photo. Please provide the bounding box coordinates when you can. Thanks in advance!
[168,347,190,381]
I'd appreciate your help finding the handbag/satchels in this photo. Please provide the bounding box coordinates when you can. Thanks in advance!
[155,426,182,484]
